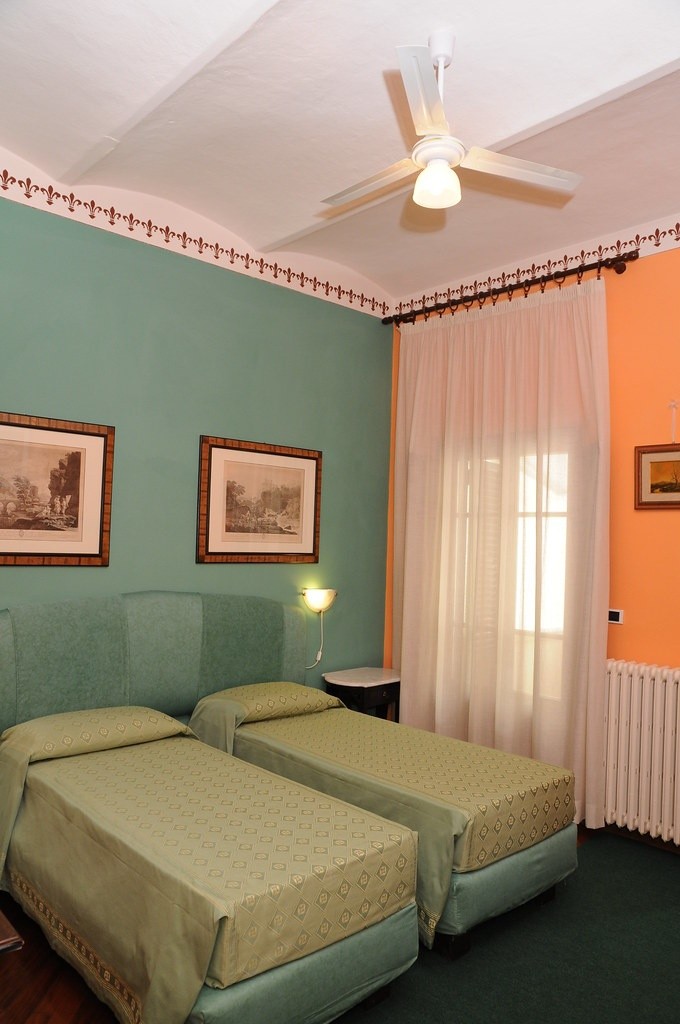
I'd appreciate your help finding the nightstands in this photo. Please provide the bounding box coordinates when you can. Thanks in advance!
[321,666,400,723]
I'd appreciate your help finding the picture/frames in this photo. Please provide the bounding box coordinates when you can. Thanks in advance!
[633,443,680,510]
[0,410,115,567]
[195,434,324,564]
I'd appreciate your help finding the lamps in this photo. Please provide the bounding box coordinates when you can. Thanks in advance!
[300,589,339,614]
[410,161,463,210]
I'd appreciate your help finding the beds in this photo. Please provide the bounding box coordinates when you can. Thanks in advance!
[0,703,428,1024]
[192,681,581,948]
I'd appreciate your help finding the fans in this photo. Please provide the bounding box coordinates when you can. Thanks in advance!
[323,33,578,220]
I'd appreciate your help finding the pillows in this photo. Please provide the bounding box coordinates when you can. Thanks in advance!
[0,706,198,771]
[188,680,349,757]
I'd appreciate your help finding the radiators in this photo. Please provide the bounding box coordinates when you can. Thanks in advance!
[602,658,680,846]
